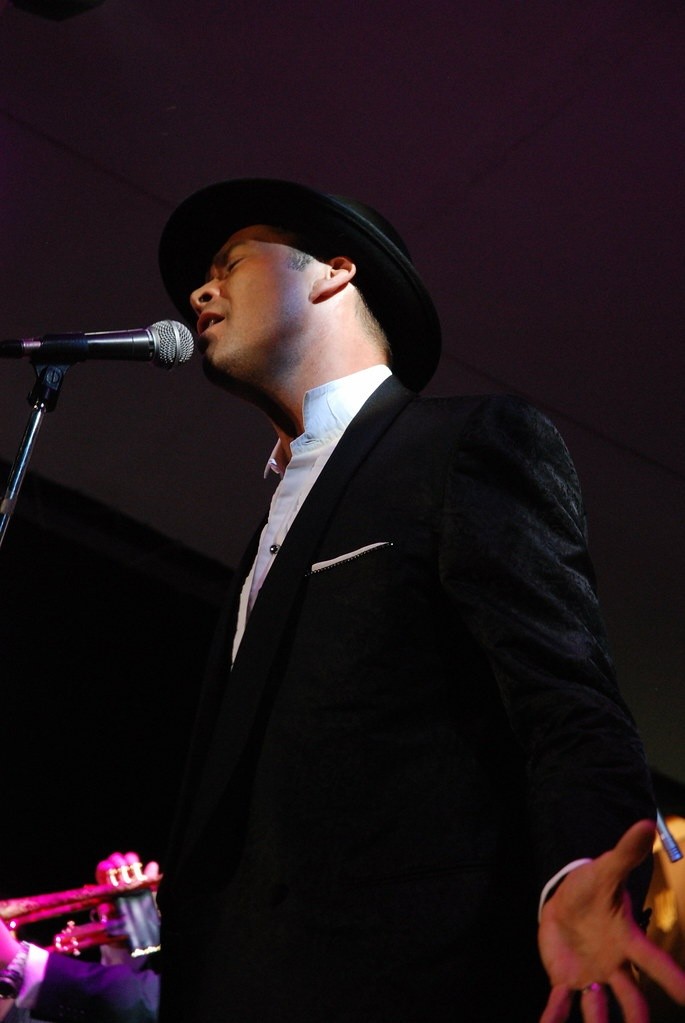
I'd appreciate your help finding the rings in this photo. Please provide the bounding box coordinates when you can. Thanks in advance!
[580,982,605,993]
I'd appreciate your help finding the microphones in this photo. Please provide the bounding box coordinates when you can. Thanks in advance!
[0,319,194,370]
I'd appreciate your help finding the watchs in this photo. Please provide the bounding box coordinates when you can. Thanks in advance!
[0,939,30,1000]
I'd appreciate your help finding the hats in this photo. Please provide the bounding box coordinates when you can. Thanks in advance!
[157,177,443,398]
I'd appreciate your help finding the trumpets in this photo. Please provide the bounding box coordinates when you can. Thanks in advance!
[0,862,164,962]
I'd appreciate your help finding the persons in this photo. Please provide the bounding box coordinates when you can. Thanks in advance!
[0,848,163,1023]
[156,177,684,1022]
